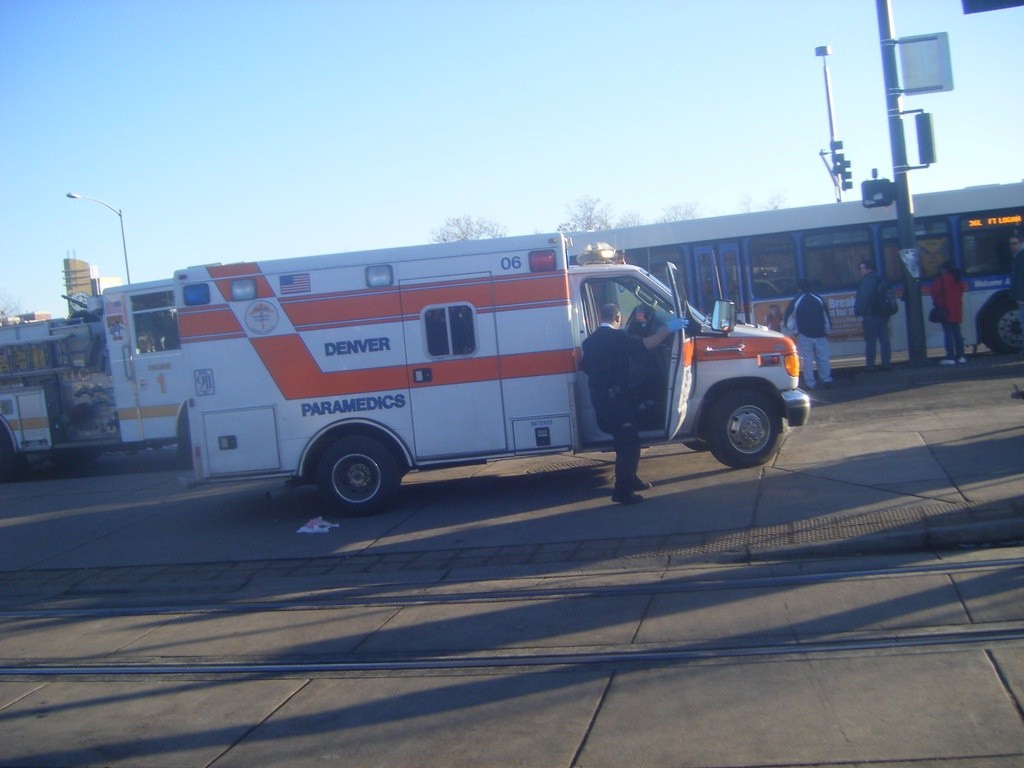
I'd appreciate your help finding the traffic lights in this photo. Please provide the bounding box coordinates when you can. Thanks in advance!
[831,141,845,174]
[840,160,853,190]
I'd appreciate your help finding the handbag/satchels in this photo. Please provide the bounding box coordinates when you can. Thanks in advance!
[928,306,948,322]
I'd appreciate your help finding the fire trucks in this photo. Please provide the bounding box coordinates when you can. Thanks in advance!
[101,232,810,517]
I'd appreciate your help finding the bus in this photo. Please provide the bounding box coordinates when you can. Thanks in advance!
[564,183,1024,371]
[0,310,111,481]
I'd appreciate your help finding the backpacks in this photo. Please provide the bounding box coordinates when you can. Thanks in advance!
[868,275,898,315]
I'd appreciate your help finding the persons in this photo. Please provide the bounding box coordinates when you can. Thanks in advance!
[854,259,891,371]
[580,303,689,503]
[767,304,783,332]
[784,277,833,389]
[1010,234,1024,334]
[931,262,966,364]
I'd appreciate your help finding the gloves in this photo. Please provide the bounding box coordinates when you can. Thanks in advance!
[665,315,689,333]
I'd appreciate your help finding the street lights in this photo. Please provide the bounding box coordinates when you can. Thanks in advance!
[66,191,132,284]
[815,45,846,203]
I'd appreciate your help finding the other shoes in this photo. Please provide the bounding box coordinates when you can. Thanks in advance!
[612,492,644,505]
[825,380,834,388]
[957,356,966,363]
[630,480,652,490]
[939,357,957,365]
